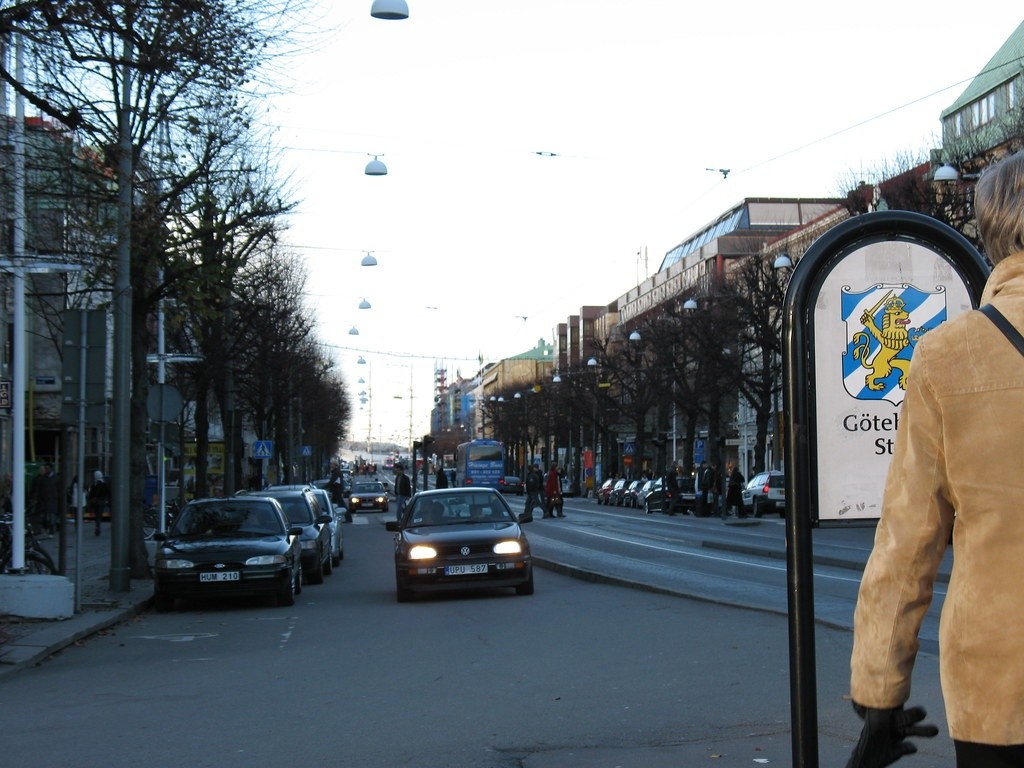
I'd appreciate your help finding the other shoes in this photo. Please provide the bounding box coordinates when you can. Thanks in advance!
[342,520,353,523]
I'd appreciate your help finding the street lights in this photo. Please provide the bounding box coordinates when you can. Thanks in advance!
[392,395,413,471]
[630,329,678,463]
[588,357,610,476]
[489,373,598,494]
[145,352,206,537]
[0,257,89,575]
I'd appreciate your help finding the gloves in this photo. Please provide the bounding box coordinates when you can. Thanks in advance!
[845,700,939,768]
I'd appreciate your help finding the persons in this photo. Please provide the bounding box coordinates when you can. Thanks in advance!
[605,470,627,480]
[354,462,375,479]
[68,470,109,536]
[31,463,61,538]
[524,462,566,518]
[640,468,655,483]
[847,153,1024,768]
[436,468,456,489]
[663,460,746,520]
[395,465,411,522]
[322,463,352,523]
[0,471,13,513]
[420,497,434,521]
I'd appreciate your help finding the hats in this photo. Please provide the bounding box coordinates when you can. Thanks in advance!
[330,457,339,466]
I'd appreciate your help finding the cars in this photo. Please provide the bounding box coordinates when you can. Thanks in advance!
[349,482,389,512]
[312,461,353,499]
[597,476,713,516]
[502,476,525,496]
[385,487,534,603]
[152,495,303,607]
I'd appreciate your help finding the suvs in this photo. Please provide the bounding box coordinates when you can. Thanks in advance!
[230,482,347,584]
[736,470,785,519]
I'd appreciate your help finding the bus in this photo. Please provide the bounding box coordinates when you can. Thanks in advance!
[453,439,506,487]
[432,453,454,482]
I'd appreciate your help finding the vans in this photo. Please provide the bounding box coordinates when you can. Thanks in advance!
[383,457,395,469]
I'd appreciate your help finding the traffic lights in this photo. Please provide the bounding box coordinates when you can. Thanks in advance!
[542,447,548,462]
[426,437,435,444]
[415,442,422,450]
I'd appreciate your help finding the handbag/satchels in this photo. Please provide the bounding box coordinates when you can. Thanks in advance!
[548,493,563,505]
